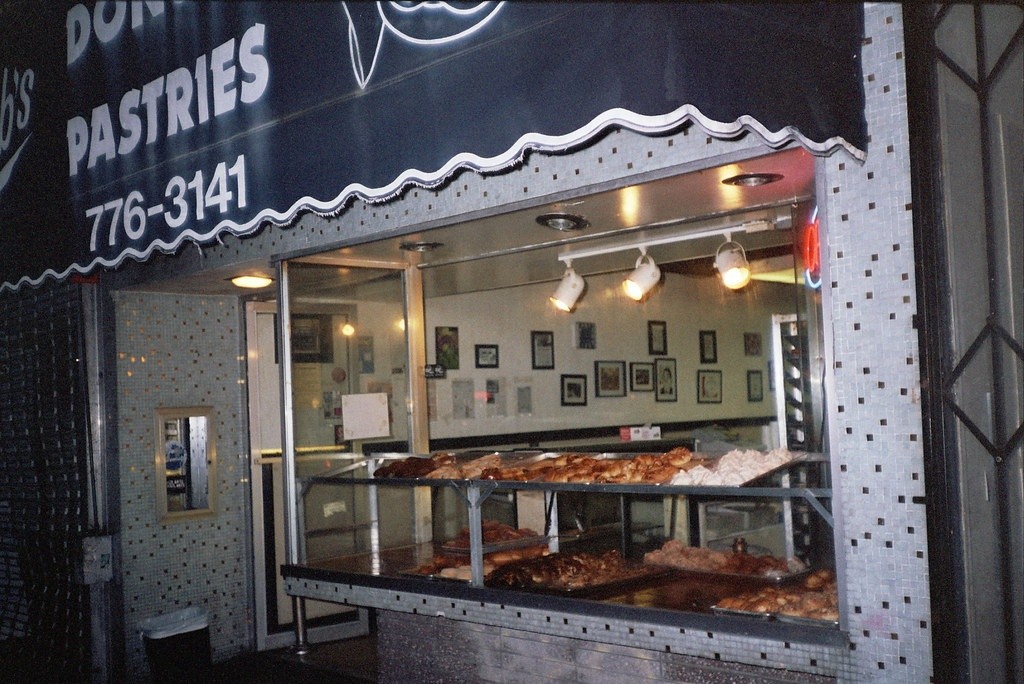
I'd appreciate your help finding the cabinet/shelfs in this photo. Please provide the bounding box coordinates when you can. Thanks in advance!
[289,449,841,645]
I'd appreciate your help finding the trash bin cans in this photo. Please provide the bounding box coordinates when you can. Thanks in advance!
[138,605,212,684]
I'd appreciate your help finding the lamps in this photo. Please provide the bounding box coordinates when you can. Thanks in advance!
[232,275,272,289]
[622,248,661,301]
[549,260,584,312]
[715,232,750,289]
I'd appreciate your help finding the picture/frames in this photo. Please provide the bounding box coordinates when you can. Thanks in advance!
[594,357,677,402]
[747,369,763,401]
[648,320,668,355]
[475,344,499,367]
[699,329,718,364]
[531,330,554,369]
[561,374,587,405]
[697,369,723,403]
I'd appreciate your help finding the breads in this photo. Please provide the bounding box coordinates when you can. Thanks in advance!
[648,539,838,625]
[417,519,548,581]
[371,446,700,486]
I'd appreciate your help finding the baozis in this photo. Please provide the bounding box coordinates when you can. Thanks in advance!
[670,447,794,486]
